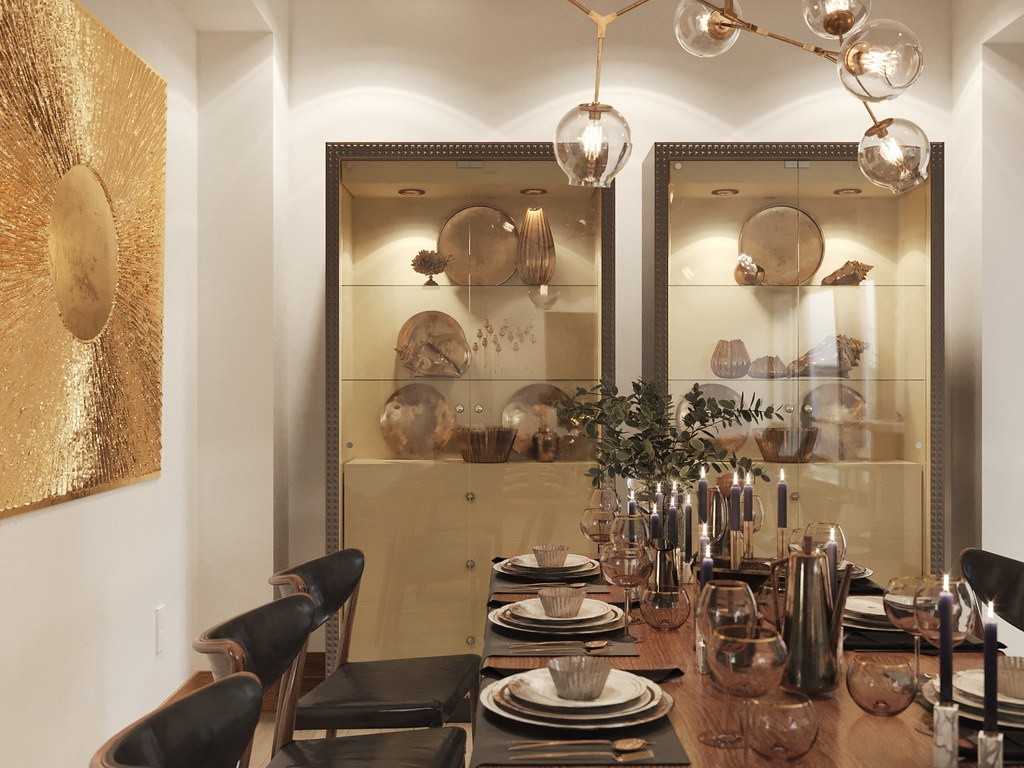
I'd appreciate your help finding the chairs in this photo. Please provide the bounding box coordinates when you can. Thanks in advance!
[269,548,481,737]
[192,593,467,768]
[89,671,264,767]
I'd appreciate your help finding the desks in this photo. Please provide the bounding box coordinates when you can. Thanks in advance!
[476,549,1023,768]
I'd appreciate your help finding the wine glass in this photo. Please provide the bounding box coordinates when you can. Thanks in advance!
[697,580,787,768]
[579,488,654,643]
[883,574,977,698]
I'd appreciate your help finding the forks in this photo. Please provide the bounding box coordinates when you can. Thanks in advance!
[509,749,655,762]
[510,647,611,655]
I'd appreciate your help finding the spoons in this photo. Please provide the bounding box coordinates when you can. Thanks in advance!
[511,582,586,589]
[508,738,648,751]
[509,640,608,648]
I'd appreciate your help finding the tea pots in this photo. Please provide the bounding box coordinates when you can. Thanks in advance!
[770,550,854,694]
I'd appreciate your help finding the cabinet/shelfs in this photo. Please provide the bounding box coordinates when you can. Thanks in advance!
[642,142,944,588]
[327,136,617,673]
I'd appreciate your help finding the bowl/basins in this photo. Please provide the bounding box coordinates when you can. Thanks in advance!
[533,544,570,567]
[538,587,587,618]
[997,656,1024,700]
[546,656,612,700]
[752,428,818,462]
[453,427,519,463]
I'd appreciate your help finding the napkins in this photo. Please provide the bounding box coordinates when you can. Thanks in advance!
[469,557,1007,768]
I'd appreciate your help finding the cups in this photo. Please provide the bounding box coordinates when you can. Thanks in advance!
[788,521,847,569]
[737,495,764,544]
[711,339,751,378]
[640,585,691,630]
[846,653,918,716]
[741,689,819,767]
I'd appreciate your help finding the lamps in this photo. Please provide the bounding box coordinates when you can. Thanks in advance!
[552,1,933,193]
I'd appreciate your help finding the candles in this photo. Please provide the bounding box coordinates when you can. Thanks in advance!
[983,598,999,736]
[938,573,953,706]
[628,466,789,610]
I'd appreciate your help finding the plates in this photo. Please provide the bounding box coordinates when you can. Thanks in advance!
[842,596,905,631]
[437,203,523,285]
[738,203,825,285]
[763,559,875,581]
[675,384,751,457]
[479,667,675,729]
[379,383,455,460]
[492,553,600,580]
[396,310,466,370]
[488,597,631,635]
[802,383,872,461]
[922,669,1024,730]
[502,383,578,458]
